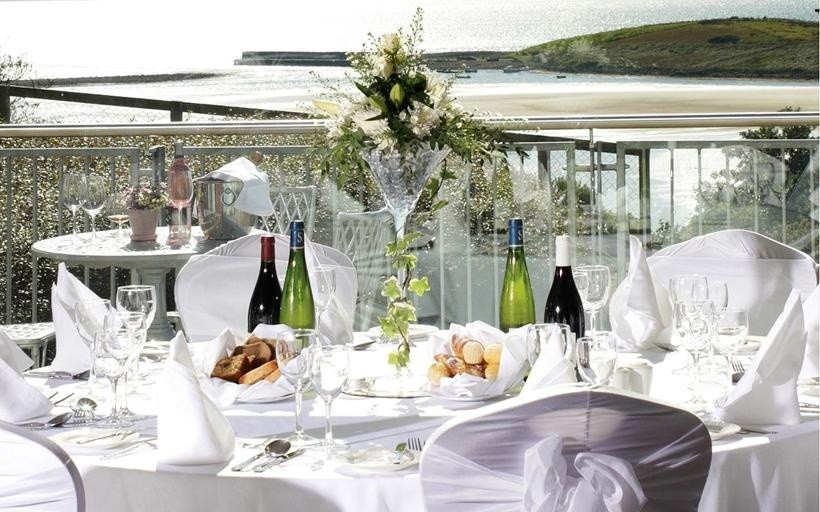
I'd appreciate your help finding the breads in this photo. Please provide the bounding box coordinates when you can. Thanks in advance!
[428,334,502,383]
[211,336,300,385]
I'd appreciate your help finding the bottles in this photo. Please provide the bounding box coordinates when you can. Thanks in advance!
[542,235,586,341]
[164,140,192,238]
[281,218,316,328]
[247,234,282,332]
[498,215,535,332]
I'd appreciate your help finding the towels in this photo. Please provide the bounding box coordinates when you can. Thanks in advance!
[187,156,278,218]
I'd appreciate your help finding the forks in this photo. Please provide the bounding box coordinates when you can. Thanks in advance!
[730,359,745,373]
[405,437,423,451]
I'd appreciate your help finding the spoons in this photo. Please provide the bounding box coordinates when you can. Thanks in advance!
[232,435,287,473]
[25,410,72,428]
[50,368,90,379]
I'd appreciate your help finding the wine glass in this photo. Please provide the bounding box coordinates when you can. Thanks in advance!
[104,190,130,238]
[667,273,749,376]
[74,285,157,427]
[276,327,322,452]
[572,264,611,332]
[167,171,197,237]
[63,172,107,244]
[304,343,352,454]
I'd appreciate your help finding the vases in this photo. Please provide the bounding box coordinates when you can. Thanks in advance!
[361,149,447,345]
[126,205,164,243]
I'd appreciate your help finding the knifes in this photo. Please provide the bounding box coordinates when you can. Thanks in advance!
[76,431,125,444]
[254,444,309,472]
[28,369,73,375]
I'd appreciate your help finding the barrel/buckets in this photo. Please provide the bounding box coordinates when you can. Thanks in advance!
[194,179,257,240]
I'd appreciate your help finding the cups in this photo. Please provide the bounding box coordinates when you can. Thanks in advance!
[527,319,653,391]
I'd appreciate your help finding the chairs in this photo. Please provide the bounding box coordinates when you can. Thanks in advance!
[252,185,318,241]
[331,206,392,334]
[2,220,820,512]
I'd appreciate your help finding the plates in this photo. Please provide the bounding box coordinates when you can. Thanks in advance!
[140,349,168,357]
[368,323,439,340]
[709,422,741,440]
[233,392,297,404]
[427,387,511,410]
[351,333,375,350]
[344,443,420,471]
[51,427,139,450]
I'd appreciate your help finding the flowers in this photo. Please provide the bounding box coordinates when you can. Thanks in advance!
[302,0,531,214]
[105,174,172,212]
